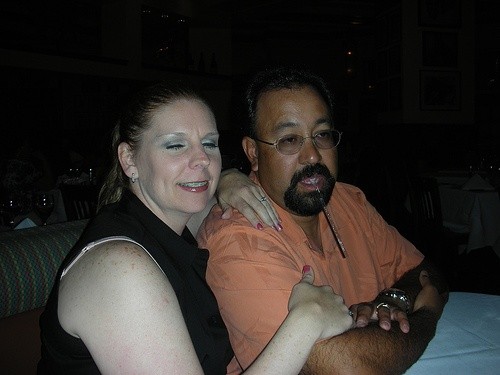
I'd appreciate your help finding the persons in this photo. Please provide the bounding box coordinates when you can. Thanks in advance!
[34,77,353,375]
[193,65,451,375]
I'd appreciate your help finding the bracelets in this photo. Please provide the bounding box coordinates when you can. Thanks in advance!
[377,288,412,315]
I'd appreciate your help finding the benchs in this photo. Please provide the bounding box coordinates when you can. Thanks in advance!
[0,218,89,375]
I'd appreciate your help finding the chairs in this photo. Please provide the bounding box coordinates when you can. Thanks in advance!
[413,179,480,245]
[59,182,99,221]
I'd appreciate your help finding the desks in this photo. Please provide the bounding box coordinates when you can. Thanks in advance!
[437,183,500,289]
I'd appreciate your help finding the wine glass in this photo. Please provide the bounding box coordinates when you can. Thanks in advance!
[33,192,56,225]
[1,190,21,231]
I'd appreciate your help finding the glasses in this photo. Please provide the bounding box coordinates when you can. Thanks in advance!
[252,129,344,156]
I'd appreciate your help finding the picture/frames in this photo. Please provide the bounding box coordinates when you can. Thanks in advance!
[375,0,476,128]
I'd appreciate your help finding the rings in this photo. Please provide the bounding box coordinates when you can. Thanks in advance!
[259,196,267,202]
[375,302,391,318]
[348,308,353,316]
